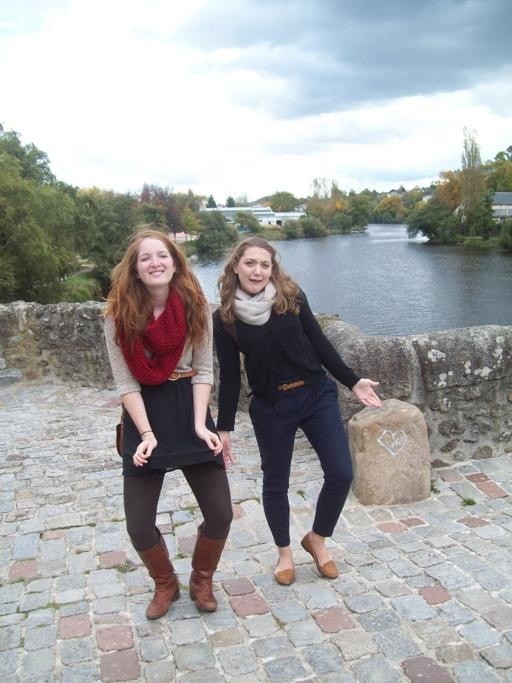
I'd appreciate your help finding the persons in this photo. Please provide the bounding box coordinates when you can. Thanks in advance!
[209,228,383,586]
[94,224,235,620]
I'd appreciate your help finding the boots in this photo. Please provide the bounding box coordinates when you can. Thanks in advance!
[188,523,229,612]
[133,524,182,620]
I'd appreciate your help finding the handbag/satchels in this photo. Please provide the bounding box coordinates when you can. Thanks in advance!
[116,416,126,457]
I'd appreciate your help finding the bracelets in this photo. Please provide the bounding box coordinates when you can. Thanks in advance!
[139,429,153,437]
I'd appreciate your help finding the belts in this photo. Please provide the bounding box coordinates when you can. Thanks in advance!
[168,369,198,380]
[275,379,305,391]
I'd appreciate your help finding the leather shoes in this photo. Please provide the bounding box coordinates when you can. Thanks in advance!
[301,532,340,579]
[274,557,296,586]
